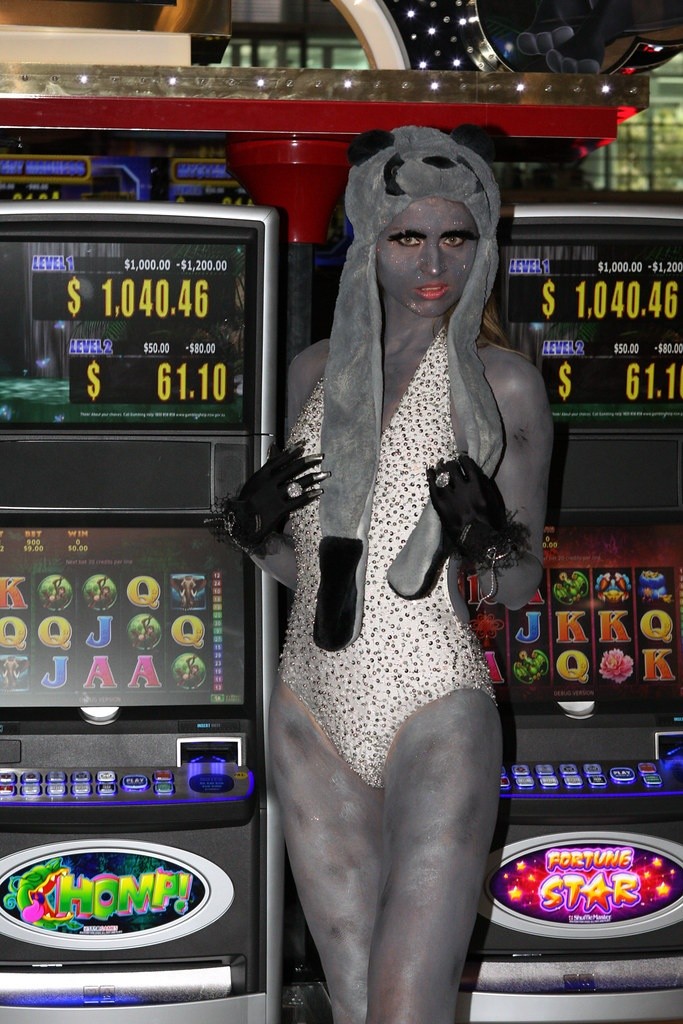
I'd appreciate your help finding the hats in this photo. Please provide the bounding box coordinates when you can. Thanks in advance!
[312,124,504,652]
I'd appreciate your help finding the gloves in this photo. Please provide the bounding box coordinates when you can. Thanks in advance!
[425,455,531,569]
[207,437,333,559]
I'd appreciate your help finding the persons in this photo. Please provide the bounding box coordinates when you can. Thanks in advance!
[203,122,556,1024]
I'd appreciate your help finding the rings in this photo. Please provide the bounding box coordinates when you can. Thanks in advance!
[286,482,302,498]
[435,471,450,488]
